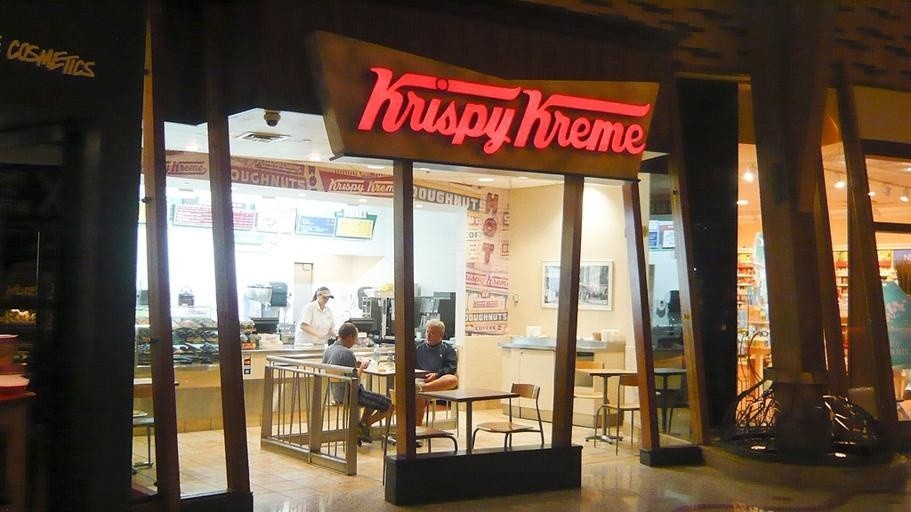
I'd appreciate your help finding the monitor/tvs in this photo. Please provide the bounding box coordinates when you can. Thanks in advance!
[349,318,374,335]
[250,317,279,334]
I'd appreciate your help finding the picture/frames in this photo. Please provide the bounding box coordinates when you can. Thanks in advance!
[541,259,615,312]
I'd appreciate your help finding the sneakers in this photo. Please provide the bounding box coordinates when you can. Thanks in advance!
[357,423,372,447]
[416,440,424,447]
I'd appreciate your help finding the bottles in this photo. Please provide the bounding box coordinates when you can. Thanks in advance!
[375,345,394,371]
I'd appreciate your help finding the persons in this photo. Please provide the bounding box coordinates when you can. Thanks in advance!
[321,321,395,446]
[414,318,460,448]
[294,286,339,344]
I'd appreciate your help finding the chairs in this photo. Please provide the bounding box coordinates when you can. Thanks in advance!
[572,359,642,456]
[325,359,546,487]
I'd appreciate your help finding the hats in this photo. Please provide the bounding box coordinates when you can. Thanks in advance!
[317,290,334,299]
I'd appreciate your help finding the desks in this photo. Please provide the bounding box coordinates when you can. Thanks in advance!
[654,367,687,433]
